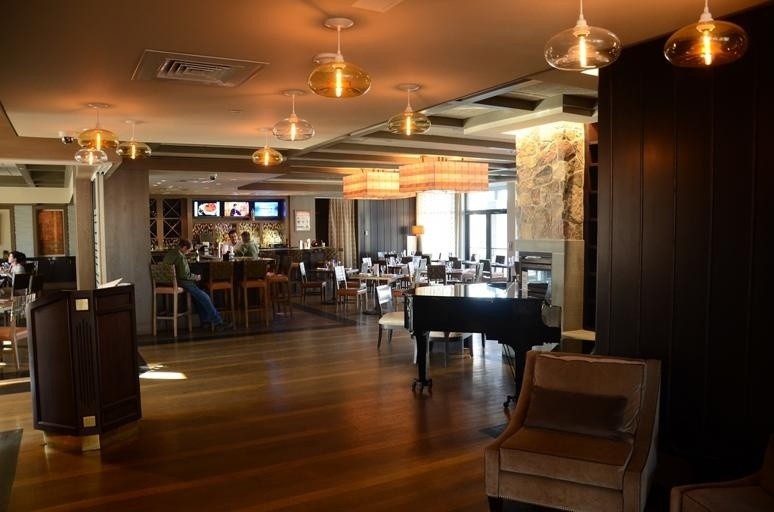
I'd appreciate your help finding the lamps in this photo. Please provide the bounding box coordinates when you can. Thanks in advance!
[75,102,152,166]
[544,0,622,72]
[411,225,424,235]
[662,0,748,66]
[252,17,431,164]
[342,173,417,200]
[398,160,489,193]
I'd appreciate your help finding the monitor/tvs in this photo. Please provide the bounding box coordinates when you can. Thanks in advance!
[252,199,286,220]
[223,201,252,217]
[194,200,223,218]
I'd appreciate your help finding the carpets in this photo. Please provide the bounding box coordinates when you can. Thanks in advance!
[0,429,23,512]
[0,335,152,397]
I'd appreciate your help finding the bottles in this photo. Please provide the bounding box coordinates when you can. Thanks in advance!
[194,248,199,263]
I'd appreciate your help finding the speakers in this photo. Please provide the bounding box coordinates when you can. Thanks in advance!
[26,285,143,436]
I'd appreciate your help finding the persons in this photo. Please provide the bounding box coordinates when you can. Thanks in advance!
[222,229,243,258]
[163,239,233,331]
[231,204,241,217]
[235,231,259,258]
[0,250,27,297]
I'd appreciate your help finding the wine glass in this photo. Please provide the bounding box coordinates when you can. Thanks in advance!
[380,266,387,277]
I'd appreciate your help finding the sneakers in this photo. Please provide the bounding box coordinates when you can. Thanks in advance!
[214,321,233,332]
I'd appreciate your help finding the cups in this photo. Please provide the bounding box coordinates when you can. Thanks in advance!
[454,284,461,297]
[322,242,325,248]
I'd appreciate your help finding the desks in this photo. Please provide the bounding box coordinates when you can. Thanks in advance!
[409,278,561,410]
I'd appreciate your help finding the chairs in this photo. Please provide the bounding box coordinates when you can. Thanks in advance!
[483,349,663,512]
[0,259,38,369]
[669,429,774,512]
